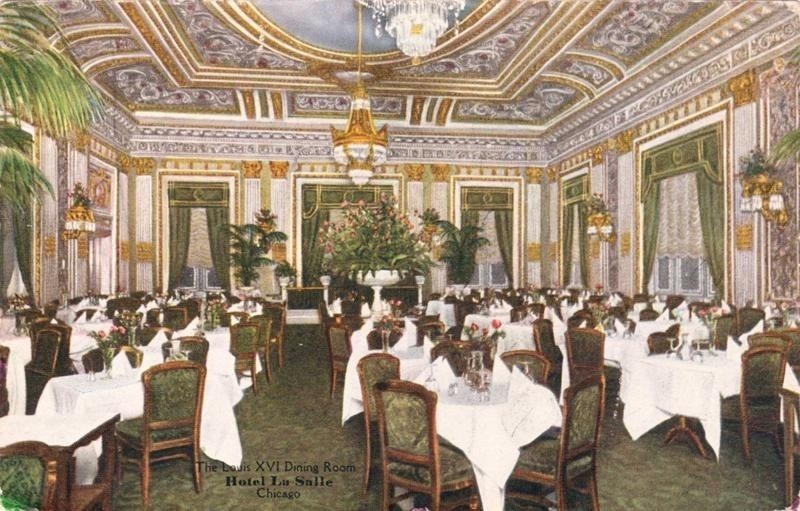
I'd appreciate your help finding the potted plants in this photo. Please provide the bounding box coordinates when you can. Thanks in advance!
[214,220,289,298]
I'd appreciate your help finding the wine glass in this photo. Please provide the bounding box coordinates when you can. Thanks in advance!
[664,337,677,362]
[677,333,692,362]
[422,364,441,395]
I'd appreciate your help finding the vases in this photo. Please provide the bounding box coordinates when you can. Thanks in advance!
[346,268,410,322]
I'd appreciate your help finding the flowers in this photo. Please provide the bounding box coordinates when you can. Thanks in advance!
[318,191,449,283]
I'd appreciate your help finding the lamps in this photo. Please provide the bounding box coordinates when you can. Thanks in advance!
[61,201,96,241]
[330,0,389,186]
[739,174,788,226]
[585,212,617,248]
[357,0,467,58]
